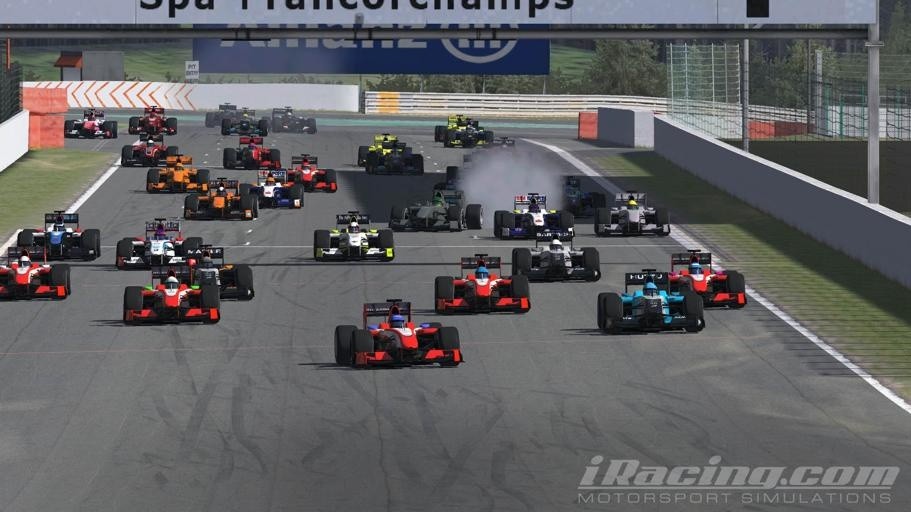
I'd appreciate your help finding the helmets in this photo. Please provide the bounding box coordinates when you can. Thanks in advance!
[164,276,179,289]
[390,315,404,328]
[629,200,638,209]
[19,255,29,268]
[147,140,154,147]
[529,204,540,212]
[200,250,212,262]
[690,263,702,274]
[476,267,488,278]
[156,224,164,234]
[643,282,658,296]
[350,222,359,233]
[550,239,562,251]
[54,224,64,231]
[267,176,275,186]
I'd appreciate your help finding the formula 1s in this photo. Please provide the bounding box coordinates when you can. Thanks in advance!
[357,112,675,243]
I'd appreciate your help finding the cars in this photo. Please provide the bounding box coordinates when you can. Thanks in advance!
[332,299,463,371]
